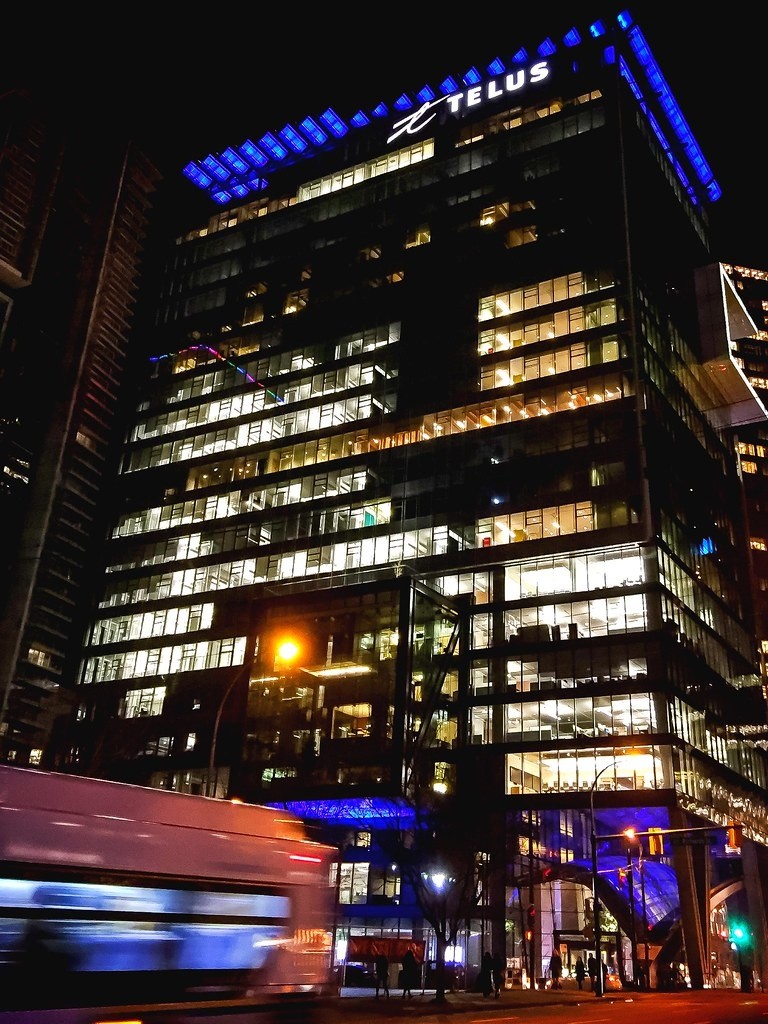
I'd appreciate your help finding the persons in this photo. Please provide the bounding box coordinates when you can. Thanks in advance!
[587,953,597,992]
[373,950,389,1000]
[549,948,562,989]
[478,948,508,1000]
[575,955,586,992]
[400,950,419,1000]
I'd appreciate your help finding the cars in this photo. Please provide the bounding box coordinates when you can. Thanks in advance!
[332,965,377,990]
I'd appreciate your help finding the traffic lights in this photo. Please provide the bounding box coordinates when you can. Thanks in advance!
[732,919,744,943]
[647,827,663,855]
[527,905,536,929]
[642,922,652,938]
[728,819,743,850]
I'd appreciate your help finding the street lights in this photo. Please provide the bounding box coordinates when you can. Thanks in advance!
[205,644,295,798]
[590,758,639,997]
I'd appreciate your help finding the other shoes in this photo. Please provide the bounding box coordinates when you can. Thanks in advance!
[373,997,379,1000]
[408,995,414,999]
[483,993,489,998]
[387,994,389,998]
[495,993,501,999]
[402,996,405,1000]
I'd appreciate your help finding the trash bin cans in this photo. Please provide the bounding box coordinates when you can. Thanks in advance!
[506,968,527,990]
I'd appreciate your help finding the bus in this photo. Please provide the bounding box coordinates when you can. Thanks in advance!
[0,764,342,1024]
